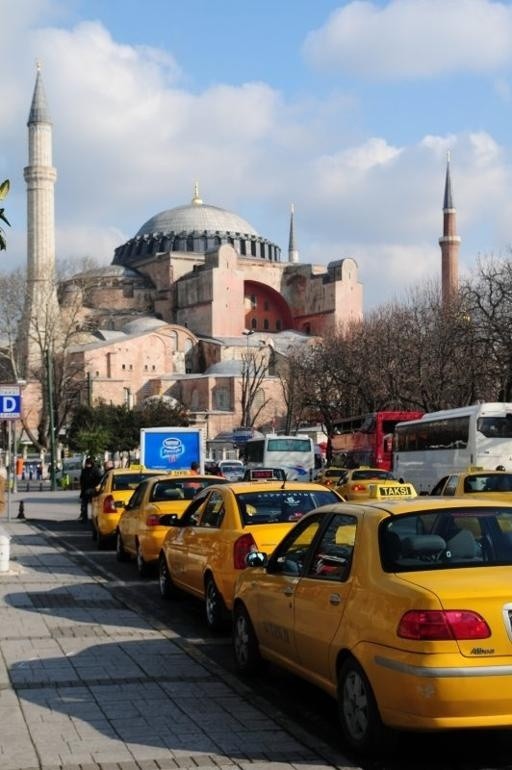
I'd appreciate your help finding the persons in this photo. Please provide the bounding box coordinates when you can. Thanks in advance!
[205,462,224,486]
[79,457,103,521]
[102,460,127,490]
[184,460,201,490]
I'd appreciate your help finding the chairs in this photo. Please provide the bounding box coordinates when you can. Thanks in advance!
[439,530,480,561]
[395,533,446,566]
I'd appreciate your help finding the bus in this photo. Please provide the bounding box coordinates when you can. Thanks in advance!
[381,402,512,497]
[328,410,425,472]
[238,434,316,482]
